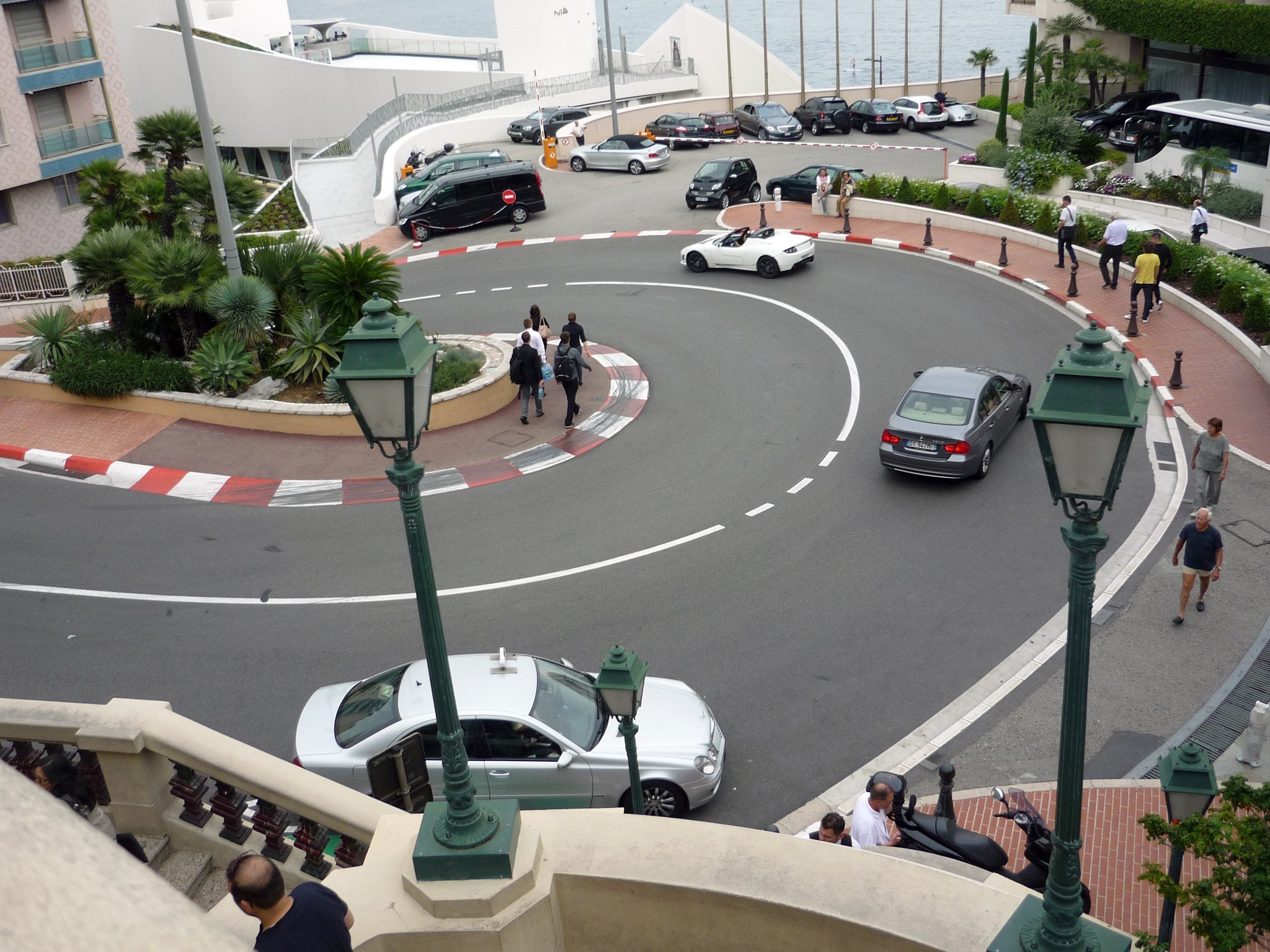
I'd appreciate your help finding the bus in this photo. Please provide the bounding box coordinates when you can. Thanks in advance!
[1133,98,1270,197]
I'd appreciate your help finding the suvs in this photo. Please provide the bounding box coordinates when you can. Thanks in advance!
[849,98,905,135]
[793,94,853,136]
[644,112,712,148]
[1073,91,1182,145]
[892,95,949,131]
[507,105,592,146]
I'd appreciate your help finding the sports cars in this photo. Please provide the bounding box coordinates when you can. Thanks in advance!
[680,226,815,279]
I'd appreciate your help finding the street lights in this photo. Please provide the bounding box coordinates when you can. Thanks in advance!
[592,645,653,815]
[989,320,1153,952]
[331,294,524,882]
[1152,742,1218,952]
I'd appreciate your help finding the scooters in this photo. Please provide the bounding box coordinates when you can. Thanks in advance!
[867,768,1092,914]
[407,143,455,169]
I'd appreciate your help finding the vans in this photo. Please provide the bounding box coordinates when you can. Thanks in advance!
[398,161,546,242]
[395,149,517,210]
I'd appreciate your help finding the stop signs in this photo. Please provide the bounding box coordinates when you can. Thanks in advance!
[503,190,517,204]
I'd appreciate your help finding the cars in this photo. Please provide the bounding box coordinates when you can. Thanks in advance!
[733,100,803,142]
[568,134,671,175]
[879,366,1031,485]
[296,641,728,819]
[698,111,742,139]
[684,156,761,209]
[766,163,870,203]
[1108,115,1141,150]
[936,96,979,126]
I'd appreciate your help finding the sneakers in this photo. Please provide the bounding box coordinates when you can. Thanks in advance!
[1124,313,1149,323]
[1150,300,1163,312]
[537,411,545,417]
[531,390,546,400]
[1190,506,1213,517]
[520,416,529,424]
[1102,279,1118,290]
[565,422,575,428]
[575,404,581,415]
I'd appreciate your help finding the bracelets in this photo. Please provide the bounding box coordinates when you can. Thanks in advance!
[1222,471,1225,474]
[1215,566,1221,571]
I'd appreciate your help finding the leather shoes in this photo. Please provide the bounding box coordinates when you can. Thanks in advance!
[1172,616,1184,624]
[1196,599,1205,612]
[1054,260,1078,268]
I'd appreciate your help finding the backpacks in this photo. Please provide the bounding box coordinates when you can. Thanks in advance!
[554,346,574,383]
[508,347,523,400]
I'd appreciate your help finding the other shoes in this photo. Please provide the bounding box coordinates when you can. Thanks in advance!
[817,197,821,203]
[824,212,828,217]
[835,213,846,219]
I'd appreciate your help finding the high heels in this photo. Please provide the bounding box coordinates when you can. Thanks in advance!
[579,382,583,386]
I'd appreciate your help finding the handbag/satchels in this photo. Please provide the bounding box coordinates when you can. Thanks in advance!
[538,316,552,339]
[1194,222,1208,236]
[541,362,555,382]
[1074,224,1077,237]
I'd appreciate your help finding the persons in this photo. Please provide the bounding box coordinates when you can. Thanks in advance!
[1188,417,1230,518]
[561,311,592,387]
[222,848,354,952]
[273,26,350,58]
[1189,200,1208,246]
[798,812,861,849]
[816,167,833,217]
[1054,196,1080,269]
[553,332,592,429]
[508,331,545,424]
[849,782,900,849]
[516,318,547,399]
[1147,233,1173,313]
[835,170,856,219]
[33,755,118,846]
[1094,210,1128,290]
[528,304,549,352]
[1123,240,1161,323]
[570,120,587,146]
[734,233,750,247]
[934,90,948,107]
[1171,509,1224,625]
